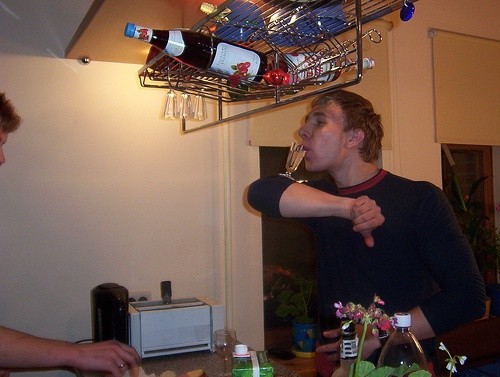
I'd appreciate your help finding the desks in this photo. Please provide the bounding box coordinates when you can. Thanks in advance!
[74,341,298,377]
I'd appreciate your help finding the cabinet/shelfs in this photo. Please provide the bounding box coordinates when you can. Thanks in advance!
[139,0,417,134]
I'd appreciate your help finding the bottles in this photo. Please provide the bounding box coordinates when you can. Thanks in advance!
[274,51,376,82]
[232,344,253,377]
[375,312,429,377]
[331,322,359,377]
[124,22,267,87]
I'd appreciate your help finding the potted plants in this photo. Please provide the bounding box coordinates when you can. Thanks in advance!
[274,275,319,359]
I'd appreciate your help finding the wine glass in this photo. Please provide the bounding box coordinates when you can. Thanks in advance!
[215,329,237,377]
[195,88,208,121]
[179,87,193,120]
[162,82,178,120]
[276,142,307,182]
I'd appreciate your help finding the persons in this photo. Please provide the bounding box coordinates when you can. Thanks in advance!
[247,90,487,367]
[0,92,140,377]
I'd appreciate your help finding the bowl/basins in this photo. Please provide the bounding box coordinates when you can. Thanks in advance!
[215,1,347,45]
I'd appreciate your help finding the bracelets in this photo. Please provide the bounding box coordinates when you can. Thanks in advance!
[378,330,388,345]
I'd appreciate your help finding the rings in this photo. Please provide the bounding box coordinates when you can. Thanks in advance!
[119,363,126,368]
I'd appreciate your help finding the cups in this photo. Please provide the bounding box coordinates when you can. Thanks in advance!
[256,351,275,377]
